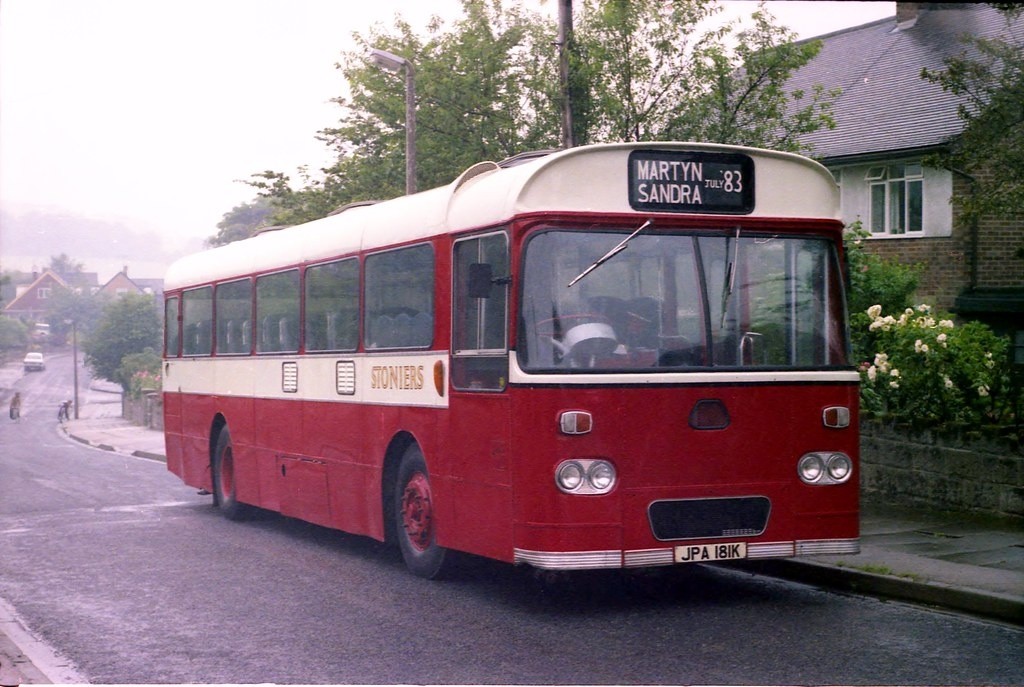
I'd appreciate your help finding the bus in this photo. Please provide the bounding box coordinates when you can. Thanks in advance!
[162,143,861,580]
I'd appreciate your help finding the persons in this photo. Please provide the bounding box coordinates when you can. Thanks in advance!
[10,392,21,419]
[57,400,72,420]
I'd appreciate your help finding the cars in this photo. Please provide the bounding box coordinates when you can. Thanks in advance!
[34,323,49,337]
[24,352,45,372]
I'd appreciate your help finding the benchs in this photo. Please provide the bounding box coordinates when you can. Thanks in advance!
[194,311,434,354]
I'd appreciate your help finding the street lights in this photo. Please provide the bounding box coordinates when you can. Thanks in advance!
[370,49,416,196]
[64,319,79,420]
